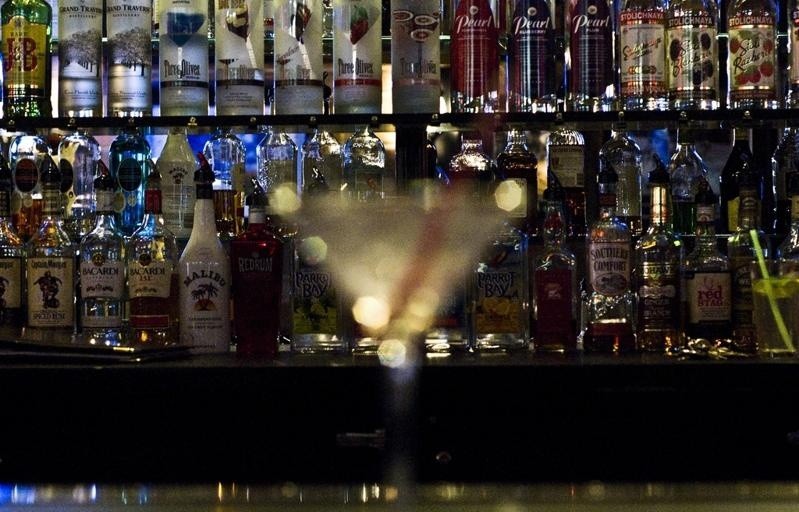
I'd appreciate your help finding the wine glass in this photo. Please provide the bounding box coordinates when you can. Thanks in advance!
[260,168,538,505]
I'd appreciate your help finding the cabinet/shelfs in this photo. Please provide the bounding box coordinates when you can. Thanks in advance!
[0,0,799,486]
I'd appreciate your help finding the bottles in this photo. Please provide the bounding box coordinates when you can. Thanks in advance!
[0,0,798,355]
